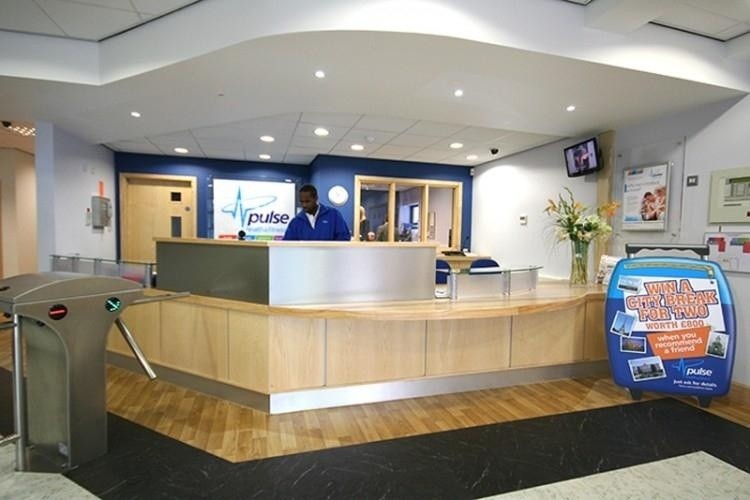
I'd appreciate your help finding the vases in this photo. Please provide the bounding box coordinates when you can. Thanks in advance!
[570,239,590,287]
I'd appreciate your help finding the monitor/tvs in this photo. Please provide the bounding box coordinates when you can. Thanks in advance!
[563,137,603,177]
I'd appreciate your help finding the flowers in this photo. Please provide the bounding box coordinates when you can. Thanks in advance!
[543,186,621,283]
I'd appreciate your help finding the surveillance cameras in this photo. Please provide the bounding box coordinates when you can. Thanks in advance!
[490,148,498,155]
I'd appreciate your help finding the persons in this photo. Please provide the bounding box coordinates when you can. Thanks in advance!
[574,144,589,171]
[642,187,666,220]
[281,183,352,241]
[360,206,399,241]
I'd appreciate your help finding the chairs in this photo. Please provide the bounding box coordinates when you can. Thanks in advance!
[436,258,500,283]
